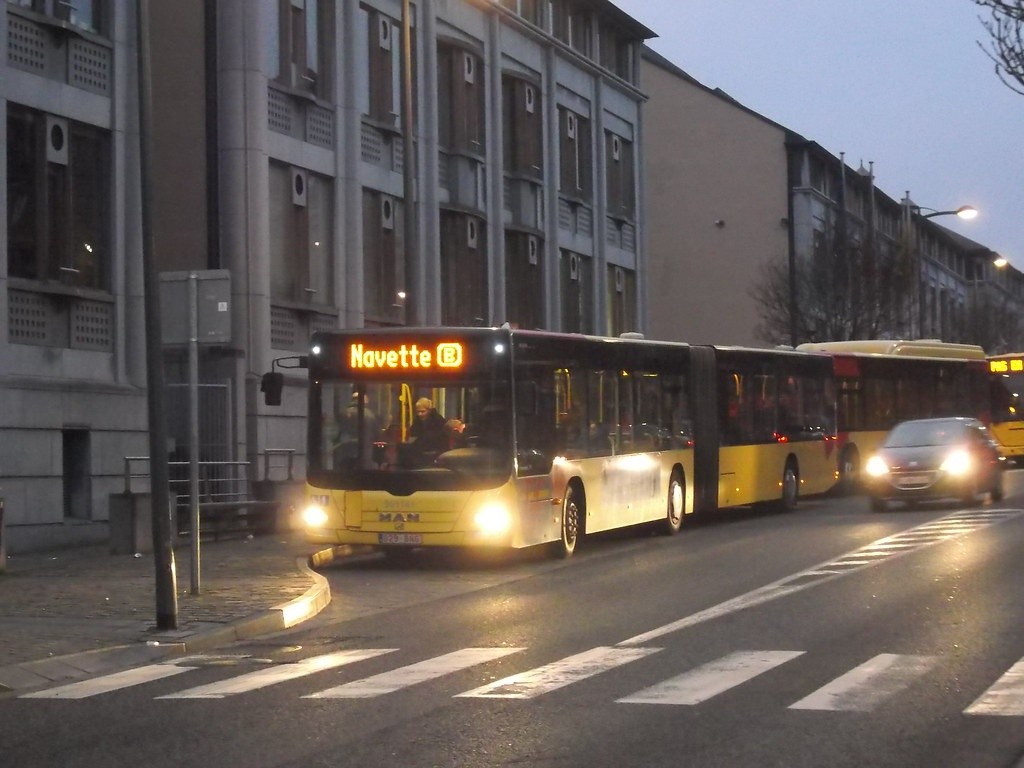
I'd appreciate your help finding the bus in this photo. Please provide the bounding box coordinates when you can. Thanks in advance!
[987,352,1023,468]
[795,339,993,490]
[719,343,842,517]
[260,324,719,556]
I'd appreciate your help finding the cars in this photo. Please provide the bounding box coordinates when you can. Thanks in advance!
[869,418,1005,509]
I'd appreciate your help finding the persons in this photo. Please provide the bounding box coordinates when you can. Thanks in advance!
[334,393,374,462]
[554,421,611,457]
[445,418,491,448]
[412,398,447,450]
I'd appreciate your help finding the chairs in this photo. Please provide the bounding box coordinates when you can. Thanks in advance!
[444,419,461,446]
[608,436,615,457]
[642,432,654,452]
